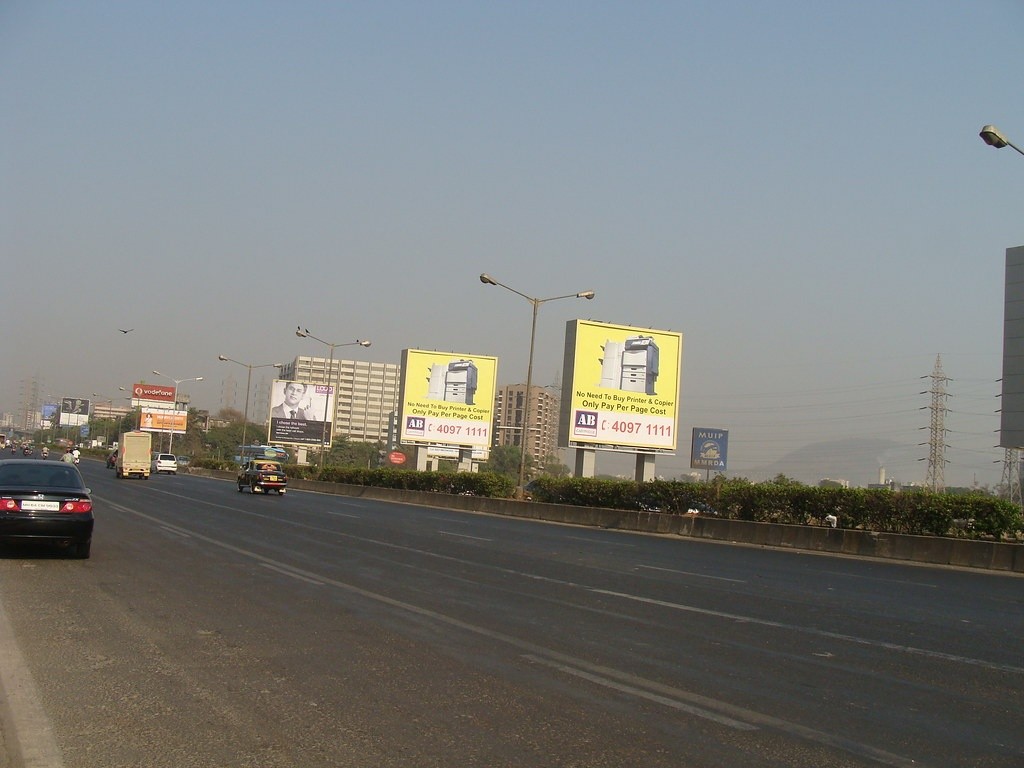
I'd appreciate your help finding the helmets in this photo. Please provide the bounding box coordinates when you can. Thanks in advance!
[67,448,73,453]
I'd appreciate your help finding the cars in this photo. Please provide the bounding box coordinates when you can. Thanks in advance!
[151,454,178,475]
[236,461,287,495]
[176,455,191,466]
[0,459,94,558]
[105,450,119,469]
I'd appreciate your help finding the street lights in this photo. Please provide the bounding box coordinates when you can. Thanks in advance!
[296,330,372,472]
[17,393,93,446]
[93,392,130,448]
[152,370,203,453]
[120,386,159,430]
[479,272,594,491]
[219,354,284,466]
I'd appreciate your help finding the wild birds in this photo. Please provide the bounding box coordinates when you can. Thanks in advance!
[118,327,134,334]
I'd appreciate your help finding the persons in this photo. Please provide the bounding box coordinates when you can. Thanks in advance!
[1,434,119,469]
[272,382,317,421]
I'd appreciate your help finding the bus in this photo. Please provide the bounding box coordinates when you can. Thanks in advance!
[233,445,290,471]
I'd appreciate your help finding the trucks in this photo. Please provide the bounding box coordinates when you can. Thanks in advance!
[116,430,152,480]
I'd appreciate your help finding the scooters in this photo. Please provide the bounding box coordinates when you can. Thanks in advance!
[1,441,80,467]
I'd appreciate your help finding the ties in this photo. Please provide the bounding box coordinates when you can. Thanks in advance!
[290,410,296,419]
